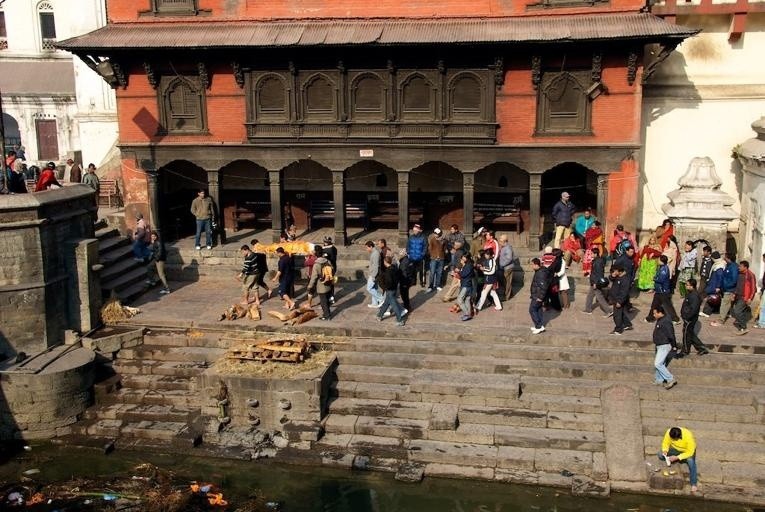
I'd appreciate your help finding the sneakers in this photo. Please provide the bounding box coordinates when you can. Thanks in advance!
[426,288,432,293]
[736,329,749,335]
[148,252,153,261]
[462,315,471,320]
[531,326,545,334]
[145,281,156,286]
[368,299,409,326]
[134,258,144,262]
[640,318,653,324]
[676,320,682,326]
[651,380,664,387]
[436,286,443,291]
[581,307,636,335]
[699,312,710,318]
[159,290,170,294]
[665,379,677,389]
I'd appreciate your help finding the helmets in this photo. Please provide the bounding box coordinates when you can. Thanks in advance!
[707,294,720,306]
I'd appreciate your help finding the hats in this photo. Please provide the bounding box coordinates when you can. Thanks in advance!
[323,236,332,243]
[135,212,143,220]
[433,227,442,237]
[413,224,423,233]
[399,248,407,256]
[561,192,570,198]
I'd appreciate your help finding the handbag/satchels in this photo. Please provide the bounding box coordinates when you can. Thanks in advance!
[598,277,609,287]
[549,283,559,295]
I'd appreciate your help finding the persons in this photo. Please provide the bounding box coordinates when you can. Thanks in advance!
[365,224,515,329]
[240,236,337,320]
[191,188,216,250]
[528,192,765,356]
[144,231,170,293]
[132,213,152,261]
[0,142,100,220]
[657,427,698,492]
[653,306,677,386]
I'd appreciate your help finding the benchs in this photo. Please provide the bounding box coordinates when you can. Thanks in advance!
[231,199,523,234]
[25,177,120,208]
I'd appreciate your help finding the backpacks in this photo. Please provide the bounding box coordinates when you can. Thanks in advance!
[1,159,14,181]
[316,260,334,288]
